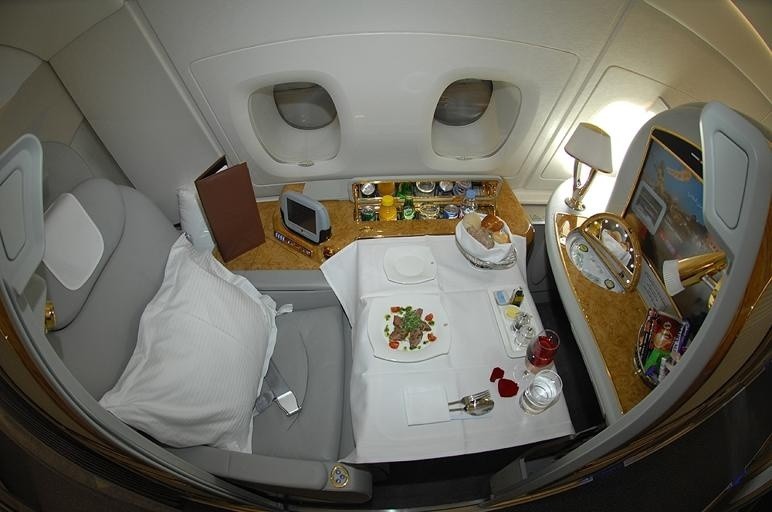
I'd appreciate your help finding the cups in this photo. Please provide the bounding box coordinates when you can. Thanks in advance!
[511,311,533,334]
[513,325,537,351]
[518,369,564,417]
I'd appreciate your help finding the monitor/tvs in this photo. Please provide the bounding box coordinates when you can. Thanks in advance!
[630,180,668,236]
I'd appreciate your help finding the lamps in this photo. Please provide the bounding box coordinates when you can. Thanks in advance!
[662,250,730,309]
[564,122,613,211]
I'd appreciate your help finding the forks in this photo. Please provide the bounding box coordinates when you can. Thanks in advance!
[448,389,492,407]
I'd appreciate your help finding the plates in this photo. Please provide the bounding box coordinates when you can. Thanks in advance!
[382,244,438,286]
[503,304,522,321]
[366,291,451,364]
[487,284,538,358]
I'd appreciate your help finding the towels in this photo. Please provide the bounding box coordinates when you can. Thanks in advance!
[403,384,451,426]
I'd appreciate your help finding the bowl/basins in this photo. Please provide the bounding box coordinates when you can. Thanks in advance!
[455,235,518,271]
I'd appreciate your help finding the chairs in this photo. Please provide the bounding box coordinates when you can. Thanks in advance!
[17,179,372,504]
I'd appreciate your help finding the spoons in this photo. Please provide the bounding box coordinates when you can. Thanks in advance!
[449,398,494,417]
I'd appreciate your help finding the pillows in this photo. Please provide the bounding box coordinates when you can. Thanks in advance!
[96,234,293,455]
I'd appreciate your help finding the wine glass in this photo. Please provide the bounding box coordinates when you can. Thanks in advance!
[513,329,561,381]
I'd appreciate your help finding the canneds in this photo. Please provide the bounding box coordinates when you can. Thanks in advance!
[361,206,376,221]
[438,181,453,196]
[361,183,376,198]
[443,205,458,218]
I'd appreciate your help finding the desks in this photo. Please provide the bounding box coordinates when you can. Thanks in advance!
[545,175,655,427]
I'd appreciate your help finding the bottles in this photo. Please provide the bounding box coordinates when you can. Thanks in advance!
[460,190,479,219]
[402,190,416,220]
[378,194,397,223]
[509,285,525,307]
